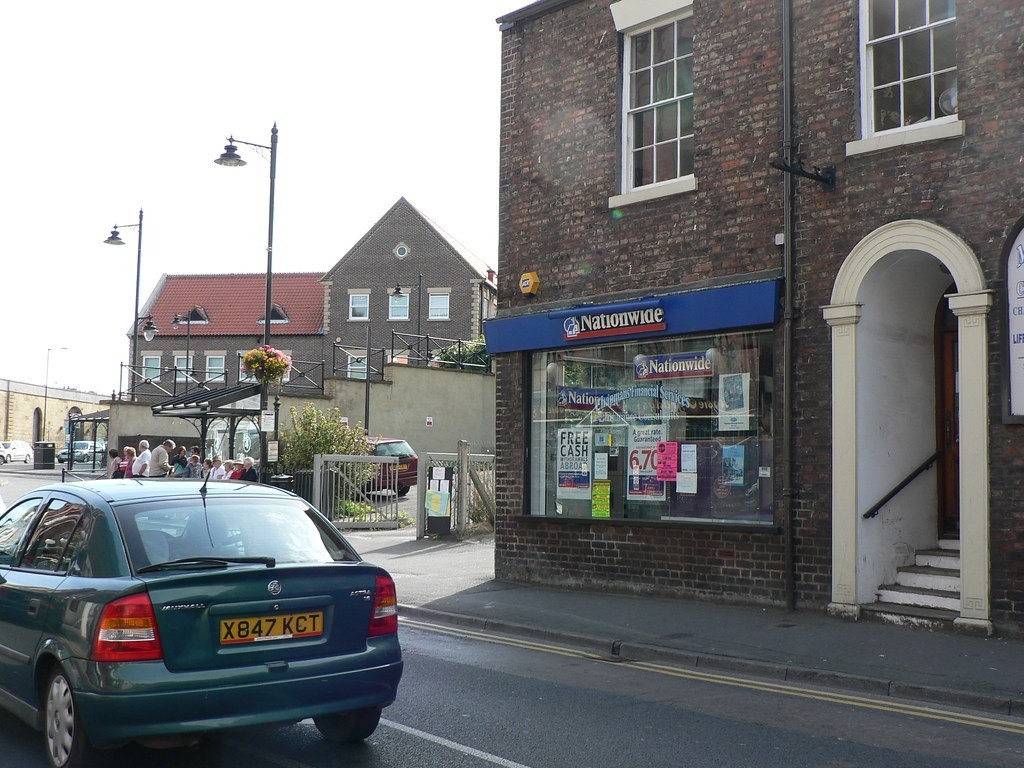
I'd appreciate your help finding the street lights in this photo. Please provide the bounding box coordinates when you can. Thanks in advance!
[389,272,423,366]
[213,121,289,482]
[102,206,157,401]
[43,347,69,442]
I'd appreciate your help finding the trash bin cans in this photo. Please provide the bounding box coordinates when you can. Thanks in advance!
[33,442,56,470]
[270,474,294,491]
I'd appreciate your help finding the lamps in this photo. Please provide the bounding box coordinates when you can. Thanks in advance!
[706,338,719,361]
[547,352,558,374]
[635,345,648,367]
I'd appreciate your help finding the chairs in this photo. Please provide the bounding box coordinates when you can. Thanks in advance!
[138,512,239,564]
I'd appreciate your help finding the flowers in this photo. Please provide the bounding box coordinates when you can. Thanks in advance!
[240,345,293,384]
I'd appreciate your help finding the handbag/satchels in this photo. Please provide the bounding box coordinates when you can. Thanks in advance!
[113,464,125,479]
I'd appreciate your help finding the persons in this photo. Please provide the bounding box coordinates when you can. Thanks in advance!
[107,439,260,483]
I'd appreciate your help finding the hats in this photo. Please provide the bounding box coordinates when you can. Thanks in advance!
[233,459,244,466]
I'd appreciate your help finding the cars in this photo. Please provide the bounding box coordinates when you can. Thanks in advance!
[0,440,34,464]
[356,437,416,496]
[55,440,104,464]
[0,478,404,768]
